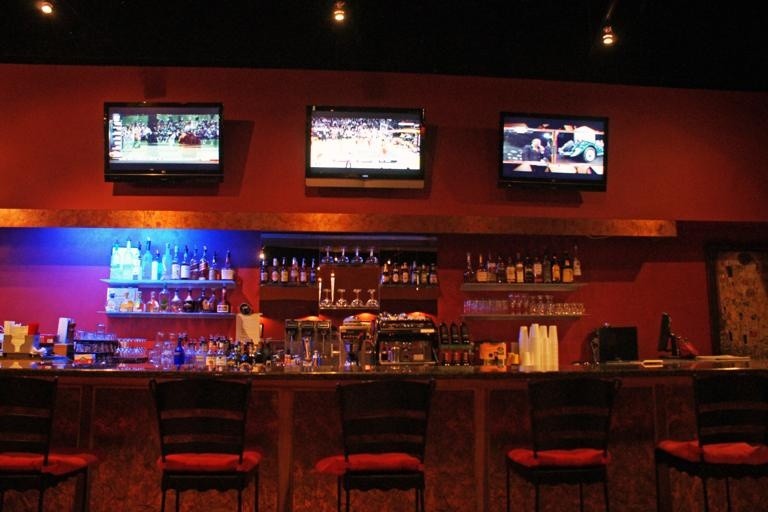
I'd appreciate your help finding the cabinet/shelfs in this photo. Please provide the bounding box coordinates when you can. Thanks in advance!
[261,235,441,352]
[460,282,591,324]
[98,277,235,320]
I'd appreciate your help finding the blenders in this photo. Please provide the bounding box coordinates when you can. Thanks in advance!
[340,320,376,372]
[285,320,333,371]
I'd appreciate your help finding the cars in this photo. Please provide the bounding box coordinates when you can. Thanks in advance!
[557,137,605,162]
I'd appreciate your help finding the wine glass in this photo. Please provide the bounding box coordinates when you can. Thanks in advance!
[320,288,379,308]
[321,245,378,265]
[507,293,555,315]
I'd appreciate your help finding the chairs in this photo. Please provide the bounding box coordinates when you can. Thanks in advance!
[505,379,621,512]
[0,375,89,512]
[148,379,260,512]
[336,379,434,512]
[656,370,767,512]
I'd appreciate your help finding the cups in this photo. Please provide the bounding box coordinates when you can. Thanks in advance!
[464,296,508,313]
[550,303,585,315]
[518,323,561,367]
[391,347,401,362]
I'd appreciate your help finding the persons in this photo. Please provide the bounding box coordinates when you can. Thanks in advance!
[126,116,217,146]
[523,138,550,174]
[313,118,422,155]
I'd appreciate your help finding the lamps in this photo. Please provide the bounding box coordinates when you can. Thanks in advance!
[39,2,54,14]
[603,21,617,51]
[332,1,346,21]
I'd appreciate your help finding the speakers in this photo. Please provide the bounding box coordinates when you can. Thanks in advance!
[594,326,638,362]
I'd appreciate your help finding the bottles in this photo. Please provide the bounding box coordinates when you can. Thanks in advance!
[284,349,321,368]
[382,347,393,362]
[463,245,582,283]
[108,235,235,314]
[260,258,439,284]
[440,322,470,344]
[78,331,286,372]
[443,352,471,366]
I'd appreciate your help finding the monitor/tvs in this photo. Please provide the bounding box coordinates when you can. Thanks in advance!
[497,111,609,191]
[307,104,425,189]
[103,101,224,183]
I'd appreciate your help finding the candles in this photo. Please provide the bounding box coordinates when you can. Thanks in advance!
[330,272,335,303]
[318,278,322,303]
